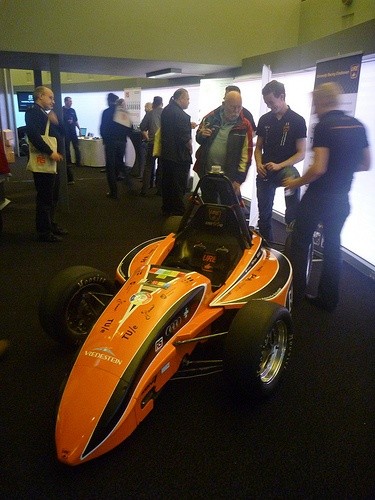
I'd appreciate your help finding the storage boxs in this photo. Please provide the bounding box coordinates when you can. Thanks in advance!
[3,129,14,163]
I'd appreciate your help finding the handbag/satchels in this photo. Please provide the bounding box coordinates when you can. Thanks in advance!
[26,113,58,174]
[111,98,132,128]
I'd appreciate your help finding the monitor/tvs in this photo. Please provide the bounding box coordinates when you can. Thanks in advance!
[79,128,86,137]
[18,91,35,111]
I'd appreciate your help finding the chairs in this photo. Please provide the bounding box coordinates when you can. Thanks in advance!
[172,203,250,289]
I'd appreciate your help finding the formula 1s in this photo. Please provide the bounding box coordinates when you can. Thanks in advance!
[42,167,314,467]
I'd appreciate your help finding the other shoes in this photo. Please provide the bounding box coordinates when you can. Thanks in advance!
[306,293,335,311]
[51,225,69,234]
[39,233,63,242]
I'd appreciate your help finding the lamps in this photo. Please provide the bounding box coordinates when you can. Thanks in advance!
[146,68,182,79]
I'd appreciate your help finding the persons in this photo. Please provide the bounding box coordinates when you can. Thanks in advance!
[194,85,257,206]
[255,80,307,246]
[101,93,131,200]
[281,83,370,312]
[25,86,68,242]
[160,89,197,214]
[63,97,84,167]
[137,96,163,196]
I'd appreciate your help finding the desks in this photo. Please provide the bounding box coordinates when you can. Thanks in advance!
[70,136,106,167]
